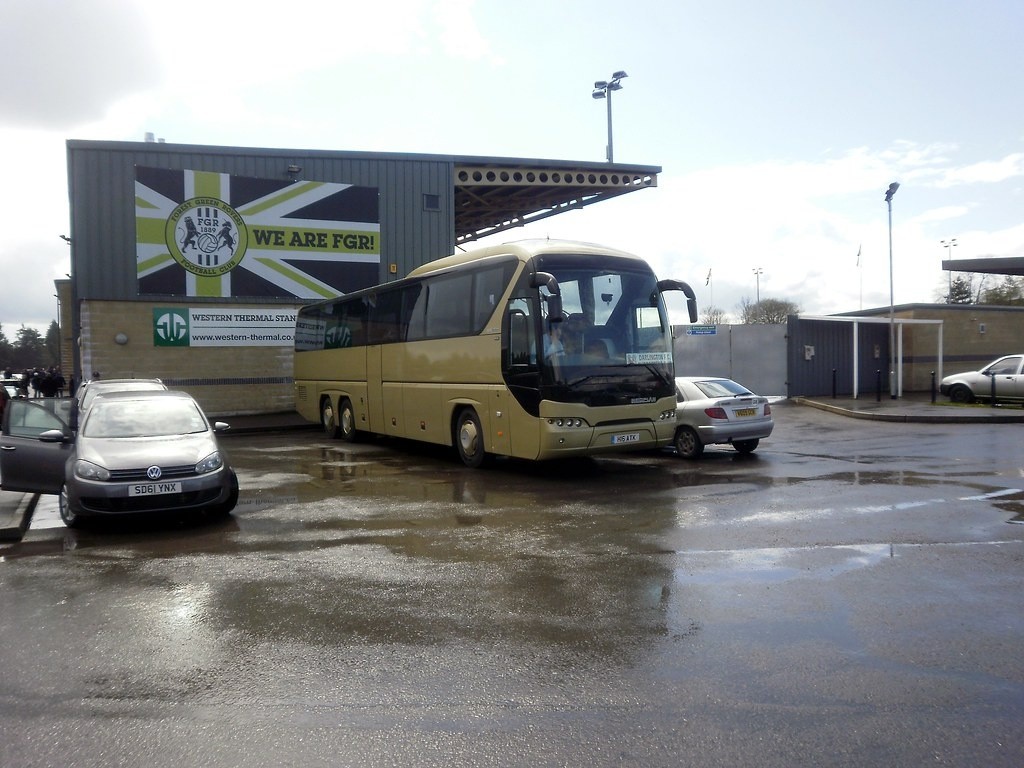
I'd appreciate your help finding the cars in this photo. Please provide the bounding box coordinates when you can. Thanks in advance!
[62,377,167,431]
[674,376,775,462]
[0,388,241,529]
[939,352,1024,402]
[5,386,26,399]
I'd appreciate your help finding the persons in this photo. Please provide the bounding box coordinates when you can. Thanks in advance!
[530,317,565,364]
[0,365,66,431]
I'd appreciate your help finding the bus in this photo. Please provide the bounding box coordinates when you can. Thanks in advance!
[291,236,699,468]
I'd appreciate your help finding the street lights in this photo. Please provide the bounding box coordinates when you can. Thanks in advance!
[753,266,764,303]
[941,237,958,303]
[590,70,627,162]
[884,183,900,400]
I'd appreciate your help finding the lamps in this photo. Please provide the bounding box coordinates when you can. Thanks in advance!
[114,333,128,344]
[287,164,301,174]
[59,234,70,241]
[65,274,71,278]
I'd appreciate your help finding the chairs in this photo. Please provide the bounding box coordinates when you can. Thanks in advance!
[513,312,611,367]
